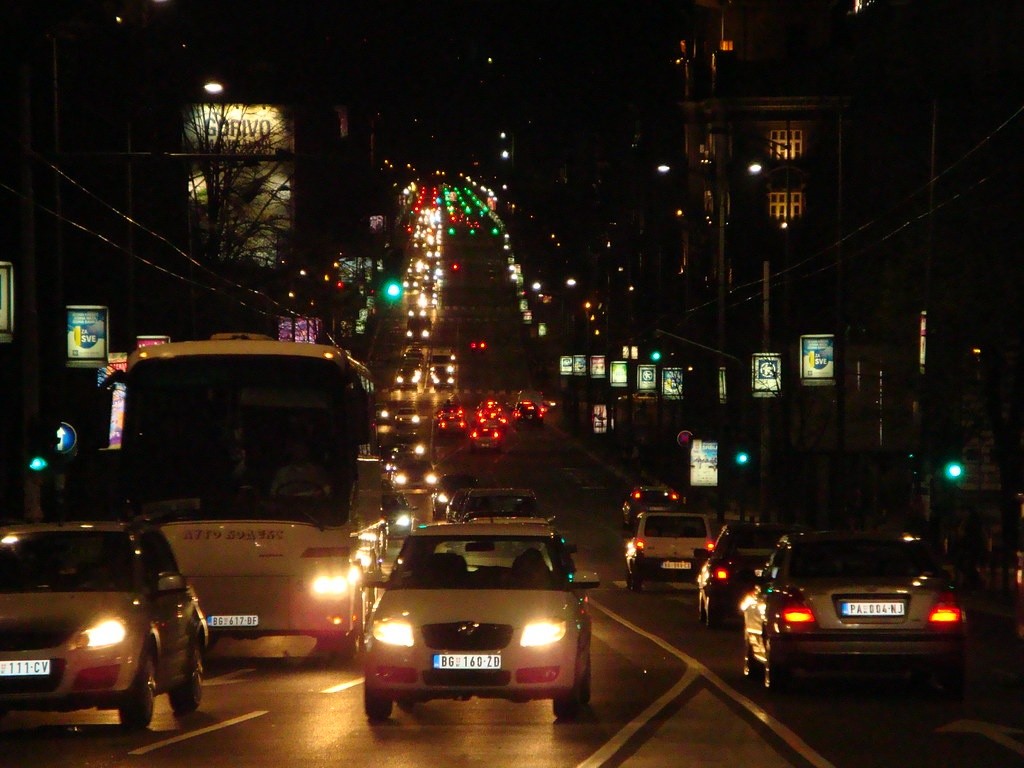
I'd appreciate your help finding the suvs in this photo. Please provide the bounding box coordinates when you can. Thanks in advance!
[621,485,684,528]
[0,519,209,733]
[691,523,790,628]
[625,513,715,592]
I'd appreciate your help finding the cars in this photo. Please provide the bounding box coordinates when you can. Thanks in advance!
[376,315,576,584]
[444,486,549,521]
[359,518,602,724]
[741,532,973,701]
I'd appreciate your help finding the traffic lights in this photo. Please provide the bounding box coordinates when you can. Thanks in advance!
[30,413,63,472]
[649,339,662,366]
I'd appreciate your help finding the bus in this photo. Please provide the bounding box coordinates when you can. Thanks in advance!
[81,331,383,663]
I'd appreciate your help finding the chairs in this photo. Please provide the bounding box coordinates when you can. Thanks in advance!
[514,547,549,581]
[424,554,464,574]
